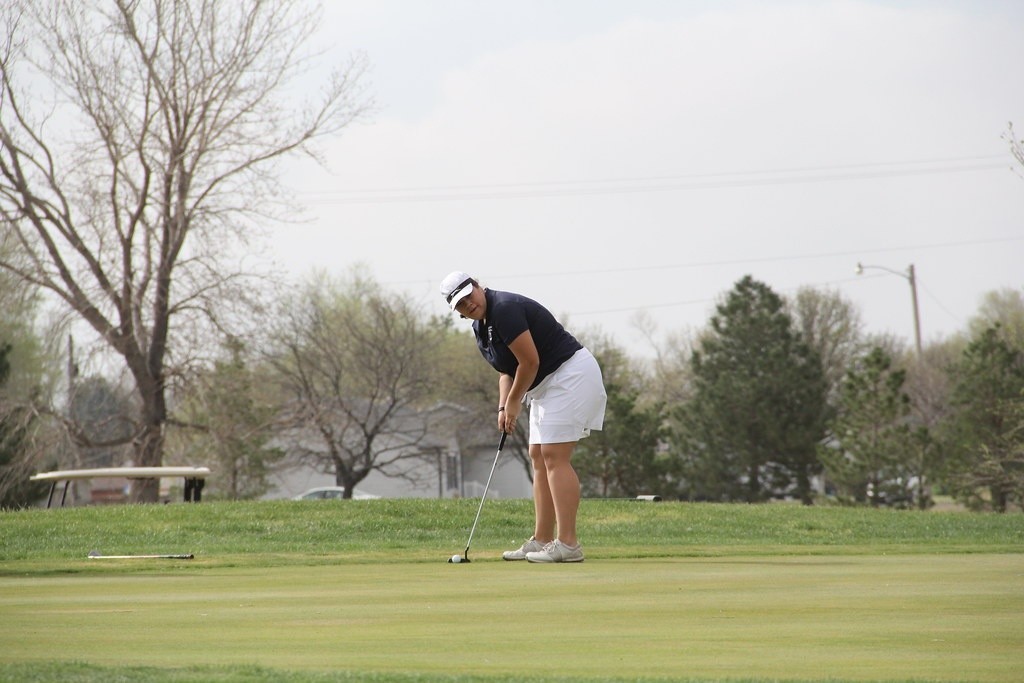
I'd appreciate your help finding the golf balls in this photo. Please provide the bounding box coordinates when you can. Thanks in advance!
[452,555,462,563]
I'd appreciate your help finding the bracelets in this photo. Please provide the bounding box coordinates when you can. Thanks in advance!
[498,407,504,412]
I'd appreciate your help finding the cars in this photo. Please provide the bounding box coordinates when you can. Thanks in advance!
[296,485,381,501]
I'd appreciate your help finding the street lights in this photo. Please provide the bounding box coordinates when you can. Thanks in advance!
[855,258,925,352]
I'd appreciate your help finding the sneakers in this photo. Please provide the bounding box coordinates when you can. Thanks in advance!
[502,536,547,561]
[525,538,584,563]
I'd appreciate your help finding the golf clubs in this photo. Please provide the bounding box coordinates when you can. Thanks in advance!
[87,549,195,560]
[447,429,507,563]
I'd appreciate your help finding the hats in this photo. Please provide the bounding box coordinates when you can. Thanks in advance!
[439,271,473,312]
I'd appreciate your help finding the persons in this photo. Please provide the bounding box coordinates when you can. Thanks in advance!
[441,270,607,564]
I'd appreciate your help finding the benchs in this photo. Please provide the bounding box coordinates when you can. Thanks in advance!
[28,467,209,509]
